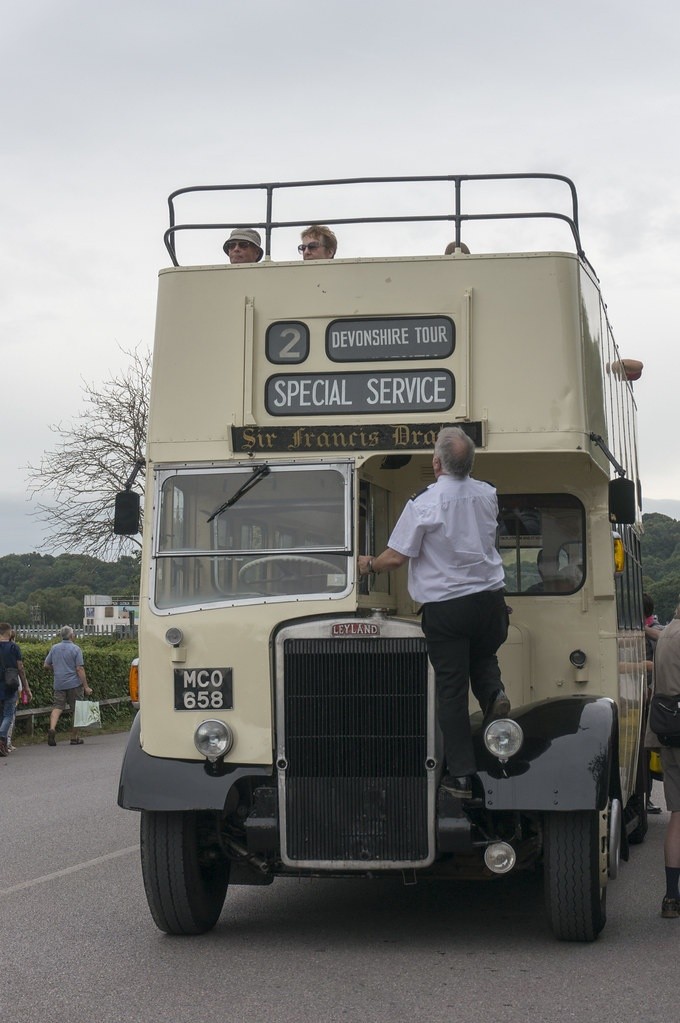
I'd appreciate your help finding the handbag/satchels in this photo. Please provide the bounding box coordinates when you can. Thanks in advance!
[73,694,102,729]
[649,694,680,746]
[5,668,19,691]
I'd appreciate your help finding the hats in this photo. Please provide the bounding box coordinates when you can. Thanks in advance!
[222,228,263,262]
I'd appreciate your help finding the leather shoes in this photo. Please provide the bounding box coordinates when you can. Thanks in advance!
[476,691,510,744]
[646,800,662,813]
[441,772,473,799]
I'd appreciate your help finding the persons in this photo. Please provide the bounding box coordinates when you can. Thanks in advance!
[223,228,264,264]
[298,225,337,260]
[524,549,575,593]
[44,626,93,746]
[358,426,511,798]
[0,622,32,756]
[642,593,680,918]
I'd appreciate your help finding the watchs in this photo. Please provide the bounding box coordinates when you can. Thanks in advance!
[367,558,377,573]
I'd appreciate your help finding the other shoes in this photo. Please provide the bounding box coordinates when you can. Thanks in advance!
[0,744,7,757]
[661,894,679,918]
[10,745,16,750]
[70,736,83,744]
[7,748,12,753]
[48,729,57,746]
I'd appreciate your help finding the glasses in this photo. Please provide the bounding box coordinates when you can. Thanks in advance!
[227,242,255,249]
[299,242,328,251]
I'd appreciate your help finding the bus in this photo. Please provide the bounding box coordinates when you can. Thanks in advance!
[116,172,648,944]
